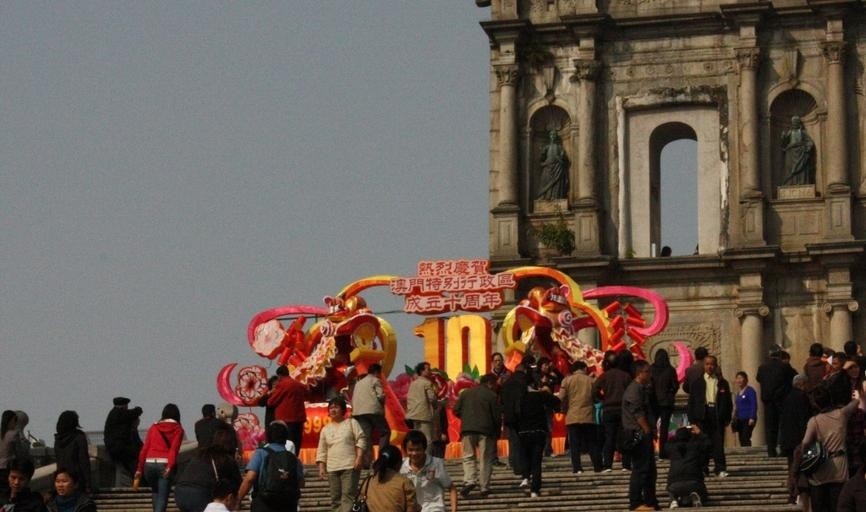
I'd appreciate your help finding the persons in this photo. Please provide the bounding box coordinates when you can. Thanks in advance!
[829,352,845,377]
[196,405,222,440]
[649,350,678,458]
[799,384,859,512]
[540,129,568,198]
[403,361,436,451]
[661,426,714,508]
[488,354,510,385]
[269,366,306,454]
[519,381,556,498]
[54,411,94,491]
[15,411,34,481]
[238,421,304,511]
[782,374,812,455]
[732,371,757,445]
[352,363,390,449]
[354,446,416,511]
[136,404,185,511]
[451,374,501,495]
[592,359,629,471]
[537,355,552,382]
[259,378,274,438]
[0,459,44,512]
[805,344,826,382]
[844,361,864,471]
[174,430,244,512]
[204,478,240,512]
[687,355,733,476]
[316,396,365,512]
[501,363,537,478]
[554,361,605,473]
[683,348,722,397]
[780,117,814,188]
[842,342,865,361]
[758,342,795,456]
[0,409,21,496]
[400,429,457,512]
[621,360,663,511]
[103,396,145,476]
[44,467,95,511]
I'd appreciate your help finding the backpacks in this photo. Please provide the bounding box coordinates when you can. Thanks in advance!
[258,447,297,501]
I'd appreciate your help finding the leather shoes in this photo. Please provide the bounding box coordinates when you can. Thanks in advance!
[649,503,664,511]
[636,503,656,510]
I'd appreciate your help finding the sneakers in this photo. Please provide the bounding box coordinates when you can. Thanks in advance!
[460,484,475,496]
[720,471,730,478]
[691,492,703,508]
[573,469,583,473]
[520,478,530,488]
[480,488,492,495]
[669,500,680,509]
[599,466,612,473]
[530,492,539,497]
[623,468,631,475]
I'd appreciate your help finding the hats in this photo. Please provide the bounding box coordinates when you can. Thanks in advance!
[113,396,131,405]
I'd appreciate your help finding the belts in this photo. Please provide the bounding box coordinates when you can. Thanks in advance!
[707,402,716,408]
[146,458,169,464]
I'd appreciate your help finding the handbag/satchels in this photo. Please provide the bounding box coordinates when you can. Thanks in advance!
[349,419,370,469]
[348,476,372,512]
[798,416,828,473]
[616,384,648,454]
